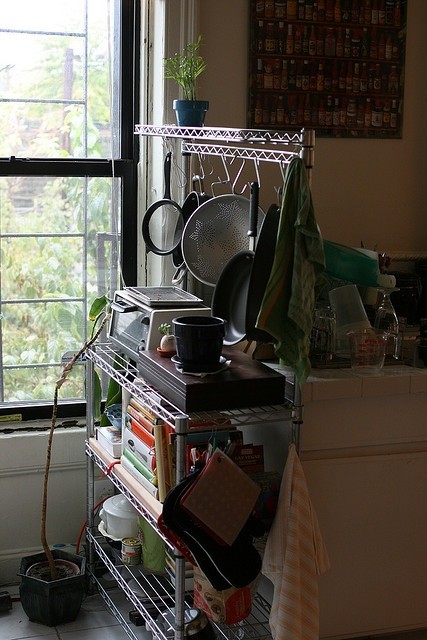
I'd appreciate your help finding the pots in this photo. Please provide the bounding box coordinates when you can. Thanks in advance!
[98,493,140,538]
[180,193,267,286]
[170,175,214,286]
[209,179,262,347]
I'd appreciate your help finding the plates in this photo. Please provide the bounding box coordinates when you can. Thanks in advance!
[170,356,227,379]
[122,283,206,307]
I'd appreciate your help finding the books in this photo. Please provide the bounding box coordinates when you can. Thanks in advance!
[95,426,122,459]
[120,396,244,504]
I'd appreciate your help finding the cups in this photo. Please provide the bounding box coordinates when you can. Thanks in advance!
[345,328,402,379]
[327,284,372,341]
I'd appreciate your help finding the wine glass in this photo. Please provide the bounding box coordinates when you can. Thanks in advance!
[374,284,401,362]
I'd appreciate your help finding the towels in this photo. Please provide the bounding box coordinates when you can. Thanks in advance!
[254,154,332,395]
[259,442,332,640]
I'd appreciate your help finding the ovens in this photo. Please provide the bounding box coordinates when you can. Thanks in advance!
[104,291,214,356]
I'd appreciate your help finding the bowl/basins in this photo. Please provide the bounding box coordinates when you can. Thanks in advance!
[102,403,122,437]
[173,313,227,369]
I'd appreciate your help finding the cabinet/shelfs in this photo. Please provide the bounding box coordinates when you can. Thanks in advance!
[69,334,300,639]
[246,1,409,140]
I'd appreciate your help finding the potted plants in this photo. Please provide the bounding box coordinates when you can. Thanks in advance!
[162,33,210,135]
[14,290,127,628]
